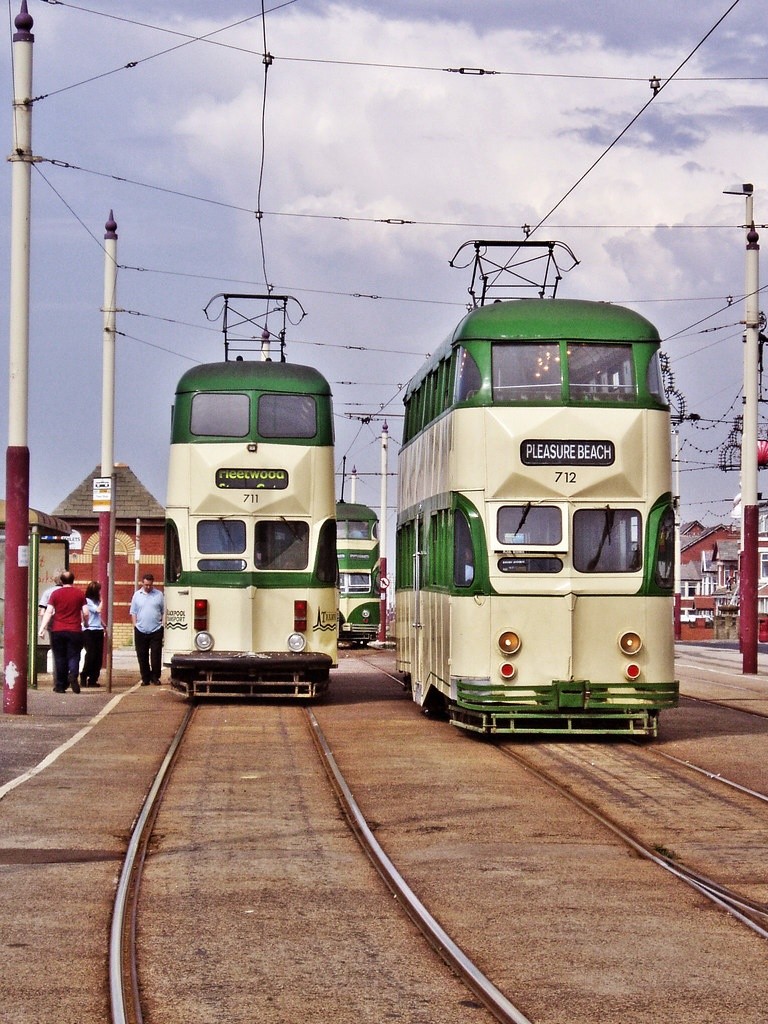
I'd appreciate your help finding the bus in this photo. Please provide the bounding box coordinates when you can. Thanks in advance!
[337,456,382,642]
[164,293,339,699]
[392,240,679,737]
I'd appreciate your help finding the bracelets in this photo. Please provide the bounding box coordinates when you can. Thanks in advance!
[104,625,107,629]
[41,624,46,630]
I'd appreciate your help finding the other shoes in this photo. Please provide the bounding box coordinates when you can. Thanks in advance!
[67,674,80,694]
[80,673,86,687]
[88,683,100,687]
[151,679,160,685]
[141,682,150,686]
[53,688,66,693]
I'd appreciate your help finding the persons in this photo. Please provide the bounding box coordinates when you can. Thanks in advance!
[129,574,164,685]
[38,568,108,694]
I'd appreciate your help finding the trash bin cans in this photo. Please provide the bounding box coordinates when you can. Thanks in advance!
[759,617,768,642]
